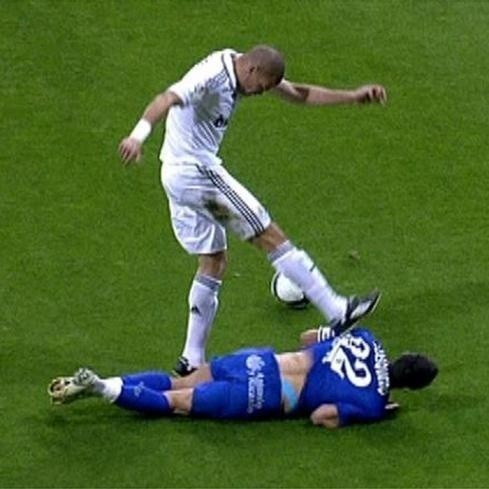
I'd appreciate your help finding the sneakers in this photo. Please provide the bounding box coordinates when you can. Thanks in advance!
[331,290,383,337]
[170,355,197,378]
[46,368,98,400]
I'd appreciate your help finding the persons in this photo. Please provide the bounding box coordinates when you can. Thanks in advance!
[117,40,391,379]
[46,323,442,433]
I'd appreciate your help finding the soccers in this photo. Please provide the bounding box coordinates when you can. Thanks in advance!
[271,271,311,309]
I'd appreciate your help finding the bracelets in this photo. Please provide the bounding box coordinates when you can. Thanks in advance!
[129,116,152,145]
